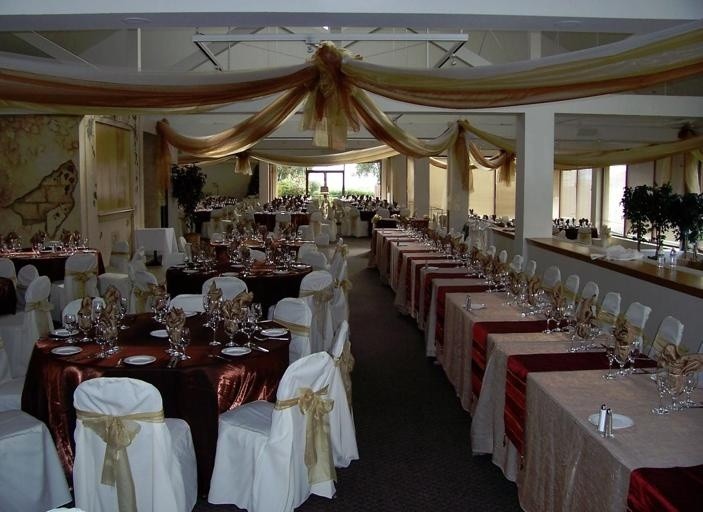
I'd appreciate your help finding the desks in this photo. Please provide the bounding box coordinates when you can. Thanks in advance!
[369,226,703,511]
[34,312,292,492]
[165,257,313,319]
[2,248,106,283]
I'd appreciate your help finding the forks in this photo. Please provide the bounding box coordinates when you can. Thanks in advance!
[253,335,289,342]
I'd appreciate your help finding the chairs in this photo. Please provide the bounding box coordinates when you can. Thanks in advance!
[0,240,147,321]
[193,191,374,241]
[159,232,350,355]
[0,182,377,512]
[0,293,350,511]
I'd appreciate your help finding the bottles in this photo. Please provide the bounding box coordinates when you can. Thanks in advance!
[597,403,613,438]
[381,228,384,234]
[669,250,677,268]
[656,249,664,267]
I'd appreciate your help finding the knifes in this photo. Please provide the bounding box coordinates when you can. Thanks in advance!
[207,353,231,362]
[167,355,179,369]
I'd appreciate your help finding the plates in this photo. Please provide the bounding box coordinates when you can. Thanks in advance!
[183,310,196,318]
[124,355,155,366]
[83,250,95,253]
[150,328,169,338]
[50,328,78,336]
[259,328,288,337]
[292,264,311,270]
[221,347,251,357]
[171,266,185,268]
[50,345,80,356]
[588,413,633,431]
[384,232,391,234]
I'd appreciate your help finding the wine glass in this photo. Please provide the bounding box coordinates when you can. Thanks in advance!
[544,295,600,351]
[650,369,696,415]
[603,332,641,380]
[0,232,88,257]
[165,325,190,360]
[396,224,544,317]
[202,290,261,349]
[182,229,302,273]
[61,296,128,358]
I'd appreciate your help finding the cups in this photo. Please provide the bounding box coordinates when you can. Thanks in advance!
[152,247,158,259]
[210,197,300,214]
[554,219,594,232]
